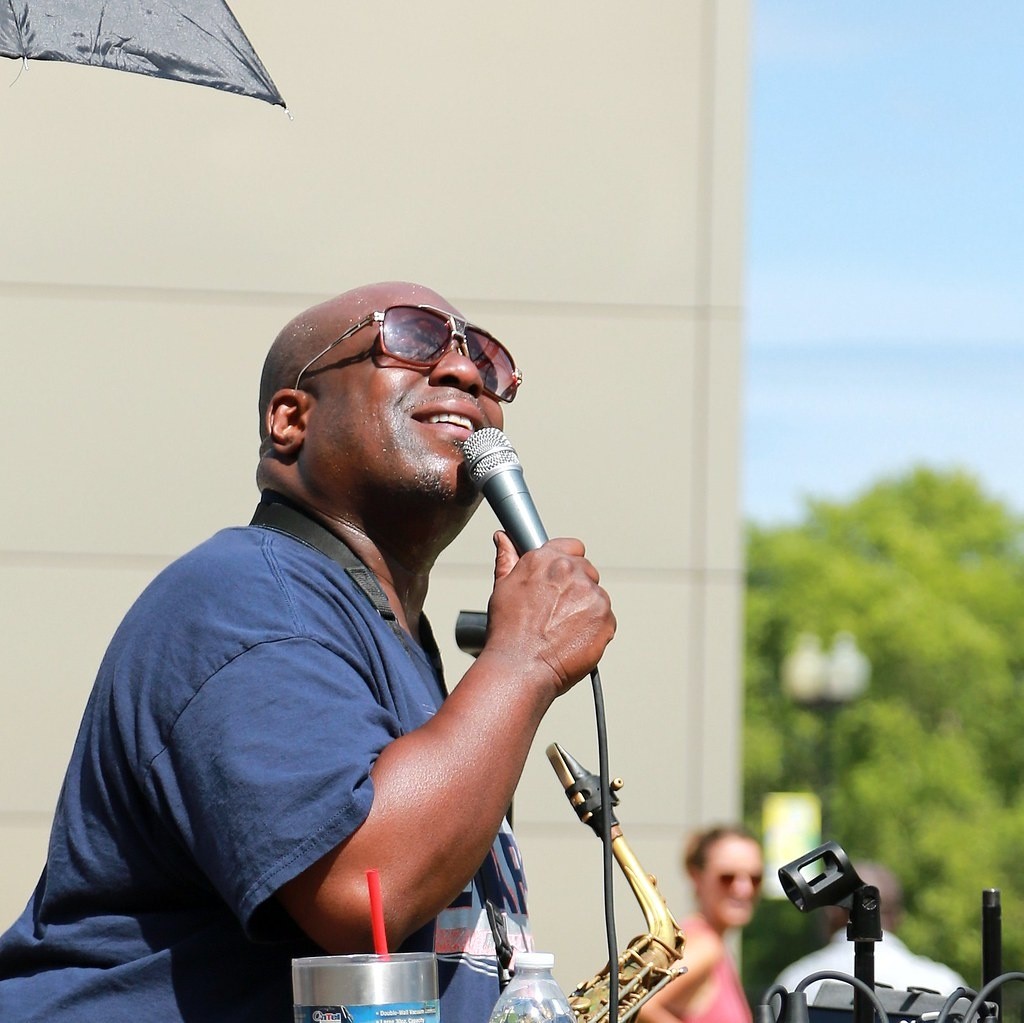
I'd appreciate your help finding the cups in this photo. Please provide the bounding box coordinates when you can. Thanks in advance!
[294,953,440,1023]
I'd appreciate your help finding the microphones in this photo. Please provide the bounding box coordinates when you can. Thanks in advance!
[459,429,549,561]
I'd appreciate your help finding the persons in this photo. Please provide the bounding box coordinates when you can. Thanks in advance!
[0,281,617,1022]
[764,862,973,1023]
[637,825,764,1023]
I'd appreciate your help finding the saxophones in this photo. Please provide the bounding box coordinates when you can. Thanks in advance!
[542,736,691,1023]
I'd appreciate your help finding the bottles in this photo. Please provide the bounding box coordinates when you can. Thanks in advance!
[489,953,577,1023]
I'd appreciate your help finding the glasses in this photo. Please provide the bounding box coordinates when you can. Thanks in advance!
[702,868,763,886]
[289,303,523,403]
